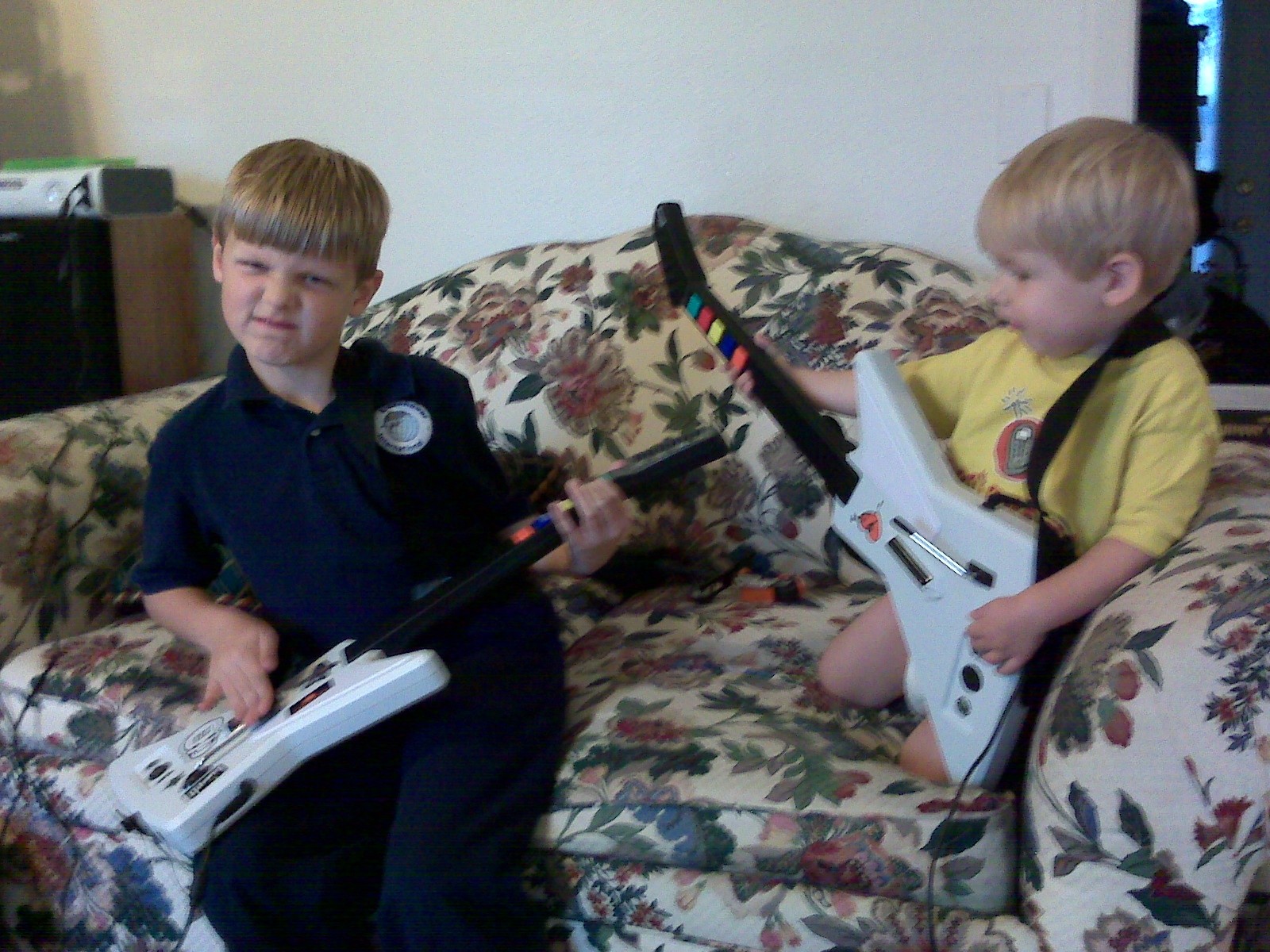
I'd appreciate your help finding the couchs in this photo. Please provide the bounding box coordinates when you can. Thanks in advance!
[0,215,1270,952]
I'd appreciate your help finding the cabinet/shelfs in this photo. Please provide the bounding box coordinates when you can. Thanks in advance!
[1,215,198,398]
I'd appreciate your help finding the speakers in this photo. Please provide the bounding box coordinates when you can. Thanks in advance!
[0,216,205,418]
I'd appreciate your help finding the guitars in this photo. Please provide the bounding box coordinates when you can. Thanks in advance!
[109,425,730,853]
[648,199,1077,794]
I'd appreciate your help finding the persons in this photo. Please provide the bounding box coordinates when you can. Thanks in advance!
[135,138,637,952]
[720,117,1219,786]
